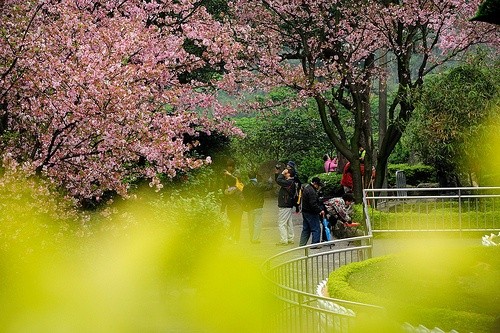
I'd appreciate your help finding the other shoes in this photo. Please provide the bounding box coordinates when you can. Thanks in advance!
[288,241,294,244]
[277,242,288,246]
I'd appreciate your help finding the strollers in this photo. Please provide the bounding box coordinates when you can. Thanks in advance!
[322,204,363,242]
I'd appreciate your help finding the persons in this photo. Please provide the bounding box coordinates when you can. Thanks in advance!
[321,194,356,245]
[242,171,274,244]
[225,178,243,245]
[286,161,301,214]
[359,146,366,160]
[299,177,323,249]
[323,154,338,173]
[275,166,295,246]
[341,161,353,192]
[220,160,235,215]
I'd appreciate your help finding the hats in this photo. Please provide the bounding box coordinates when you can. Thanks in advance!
[287,161,295,168]
[312,177,323,186]
